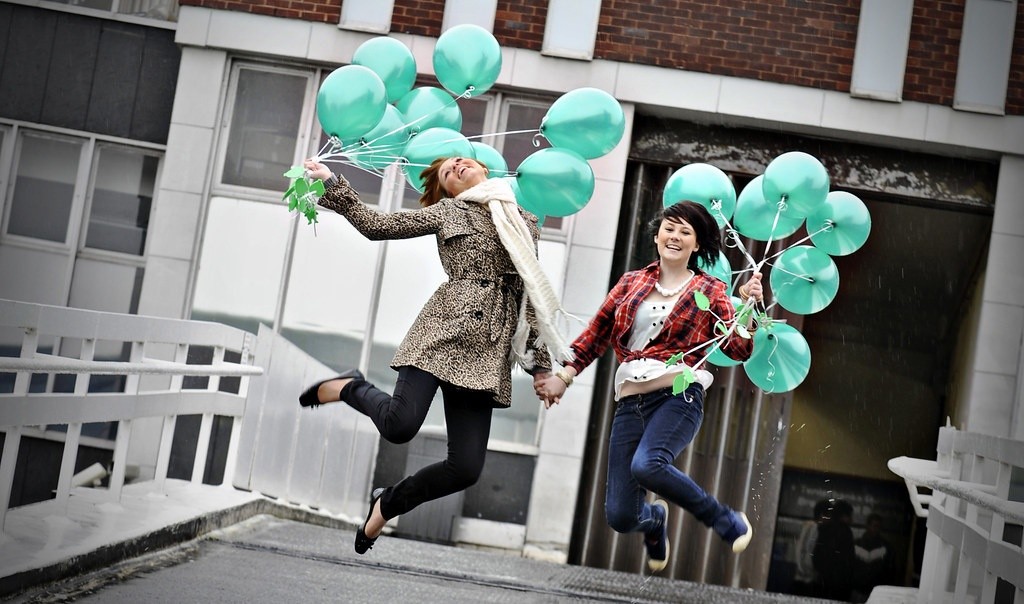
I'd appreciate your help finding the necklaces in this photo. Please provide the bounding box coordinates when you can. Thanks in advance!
[654,269,695,297]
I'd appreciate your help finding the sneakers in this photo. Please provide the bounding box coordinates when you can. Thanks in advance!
[642,500,670,571]
[726,512,753,554]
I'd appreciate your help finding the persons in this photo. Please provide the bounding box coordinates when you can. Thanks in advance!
[533,200,763,570]
[299,157,559,554]
[785,499,888,604]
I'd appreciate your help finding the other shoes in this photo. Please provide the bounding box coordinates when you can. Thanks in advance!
[355,488,385,554]
[299,368,365,408]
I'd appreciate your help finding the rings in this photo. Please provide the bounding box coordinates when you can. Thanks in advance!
[545,392,549,397]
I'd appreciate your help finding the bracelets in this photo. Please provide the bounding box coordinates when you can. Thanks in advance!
[555,369,573,387]
[739,285,763,303]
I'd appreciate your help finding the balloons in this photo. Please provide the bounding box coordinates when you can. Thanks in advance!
[508,147,595,227]
[733,151,829,241]
[351,36,417,105]
[705,296,811,393]
[401,127,476,194]
[538,87,626,160]
[342,102,409,170]
[432,24,502,99]
[469,142,509,179]
[316,65,387,140]
[395,86,463,138]
[770,191,872,315]
[662,162,737,299]
